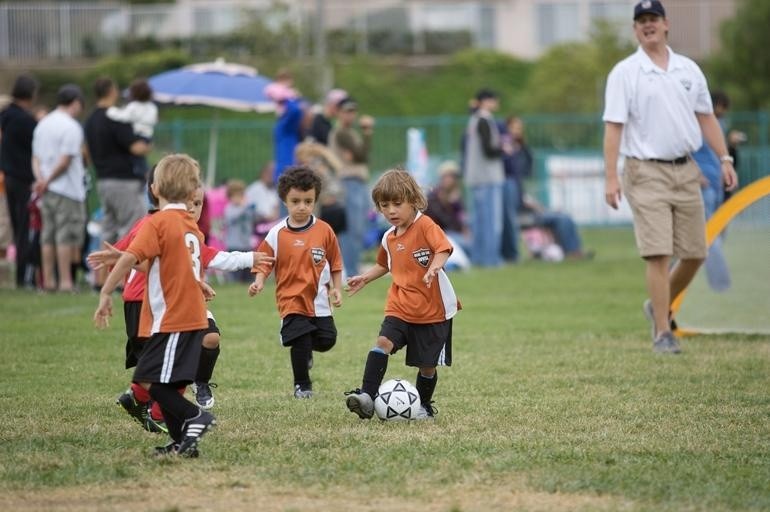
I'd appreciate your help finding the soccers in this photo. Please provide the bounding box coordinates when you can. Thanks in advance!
[374,377,420,422]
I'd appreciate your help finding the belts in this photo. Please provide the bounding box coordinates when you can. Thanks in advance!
[626,156,689,164]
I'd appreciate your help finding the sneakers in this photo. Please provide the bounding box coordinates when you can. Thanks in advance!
[119,383,219,459]
[344,388,374,418]
[308,351,313,368]
[644,299,672,338]
[417,401,438,420]
[293,384,312,399]
[652,331,681,354]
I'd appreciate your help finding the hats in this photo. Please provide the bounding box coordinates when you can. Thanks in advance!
[634,0,665,20]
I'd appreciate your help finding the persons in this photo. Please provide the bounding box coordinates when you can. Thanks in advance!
[249,165,341,400]
[342,167,462,424]
[603,1,747,357]
[94,153,276,460]
[0,76,280,294]
[264,76,594,277]
